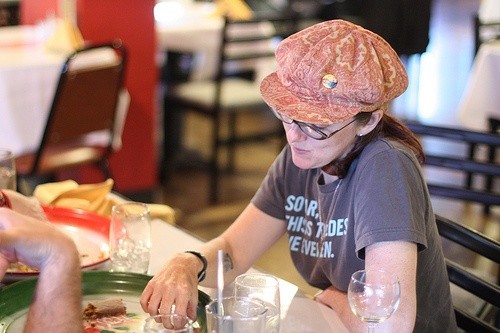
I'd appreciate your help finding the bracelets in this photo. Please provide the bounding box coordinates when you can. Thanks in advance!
[183,251,207,284]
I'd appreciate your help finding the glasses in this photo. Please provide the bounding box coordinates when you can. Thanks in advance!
[267,105,359,141]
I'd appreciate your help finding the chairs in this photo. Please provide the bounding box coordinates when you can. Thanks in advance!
[0,13,500,333]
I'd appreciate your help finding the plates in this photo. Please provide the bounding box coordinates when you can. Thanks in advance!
[0,270,232,333]
[0,207,127,280]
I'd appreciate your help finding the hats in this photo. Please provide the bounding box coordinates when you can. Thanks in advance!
[260,20,409,126]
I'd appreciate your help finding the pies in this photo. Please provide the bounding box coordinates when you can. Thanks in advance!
[82,298,127,321]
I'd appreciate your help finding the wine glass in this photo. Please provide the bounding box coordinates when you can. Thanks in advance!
[347,269,402,333]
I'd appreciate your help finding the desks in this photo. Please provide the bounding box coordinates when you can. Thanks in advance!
[97,190,301,320]
[0,20,73,195]
[152,2,276,182]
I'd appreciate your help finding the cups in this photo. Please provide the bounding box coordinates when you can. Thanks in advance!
[145,314,193,333]
[0,149,16,192]
[109,203,152,274]
[204,296,269,333]
[231,274,280,333]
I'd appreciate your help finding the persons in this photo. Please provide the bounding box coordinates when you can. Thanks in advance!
[0,188,83,333]
[139,20,458,333]
